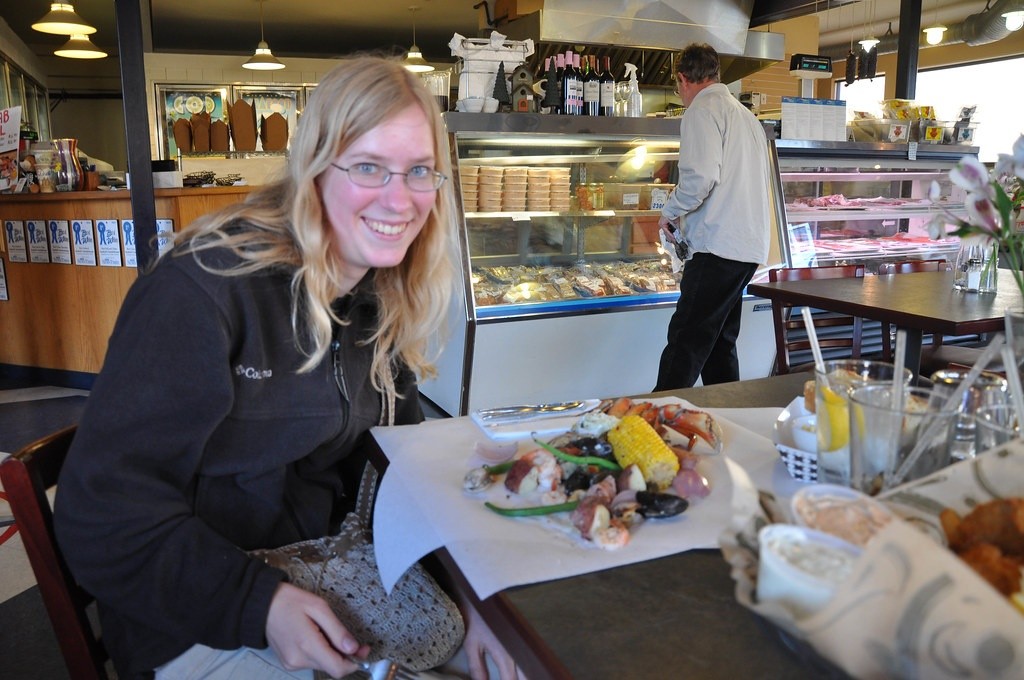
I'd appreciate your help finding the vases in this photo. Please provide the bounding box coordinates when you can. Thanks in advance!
[1005,304,1024,425]
[953,240,1000,294]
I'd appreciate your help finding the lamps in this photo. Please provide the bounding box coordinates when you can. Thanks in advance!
[404,7,435,73]
[1001,0,1024,31]
[53,34,108,60]
[858,0,880,53]
[241,0,286,71]
[922,0,948,45]
[31,0,98,36]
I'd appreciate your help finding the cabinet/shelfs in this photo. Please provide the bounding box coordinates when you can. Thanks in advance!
[775,137,981,367]
[407,114,789,418]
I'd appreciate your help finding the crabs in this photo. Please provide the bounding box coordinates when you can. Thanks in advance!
[598,399,726,457]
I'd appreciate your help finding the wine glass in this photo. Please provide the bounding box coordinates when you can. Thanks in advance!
[619,81,632,117]
[614,83,622,117]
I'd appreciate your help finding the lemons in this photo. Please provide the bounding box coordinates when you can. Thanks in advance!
[822,390,849,454]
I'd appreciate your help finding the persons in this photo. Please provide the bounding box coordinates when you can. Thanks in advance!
[54,58,528,680]
[650,43,770,393]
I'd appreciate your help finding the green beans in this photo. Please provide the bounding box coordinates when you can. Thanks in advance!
[483,431,619,517]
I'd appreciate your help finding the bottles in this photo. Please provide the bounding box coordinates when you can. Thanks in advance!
[954,240,982,292]
[542,51,615,116]
[570,196,580,211]
[589,182,604,210]
[575,182,594,210]
[49,138,84,191]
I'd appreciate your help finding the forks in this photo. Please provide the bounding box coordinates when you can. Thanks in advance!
[346,653,420,680]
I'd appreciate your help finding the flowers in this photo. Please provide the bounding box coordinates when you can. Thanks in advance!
[919,131,1023,299]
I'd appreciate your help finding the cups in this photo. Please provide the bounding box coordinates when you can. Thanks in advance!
[847,386,963,496]
[34,163,53,193]
[927,369,1008,464]
[423,72,451,112]
[814,360,912,486]
[976,405,1024,457]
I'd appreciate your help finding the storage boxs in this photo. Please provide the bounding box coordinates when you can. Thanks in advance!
[848,120,982,146]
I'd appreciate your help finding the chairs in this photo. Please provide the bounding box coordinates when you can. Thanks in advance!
[0,424,118,680]
[770,265,864,375]
[878,259,1024,378]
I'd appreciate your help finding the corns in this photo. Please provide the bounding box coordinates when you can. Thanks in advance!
[607,414,680,490]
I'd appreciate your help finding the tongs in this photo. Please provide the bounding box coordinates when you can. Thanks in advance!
[479,401,584,420]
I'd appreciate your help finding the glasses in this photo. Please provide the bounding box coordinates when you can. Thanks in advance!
[332,163,448,191]
[672,84,681,98]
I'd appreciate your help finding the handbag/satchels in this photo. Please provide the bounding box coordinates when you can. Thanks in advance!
[241,514,467,672]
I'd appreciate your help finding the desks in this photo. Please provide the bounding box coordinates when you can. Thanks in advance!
[747,268,1024,396]
[366,371,1019,680]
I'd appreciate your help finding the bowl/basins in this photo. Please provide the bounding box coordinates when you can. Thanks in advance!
[456,102,467,112]
[757,523,868,607]
[463,99,484,113]
[792,414,817,452]
[483,99,499,113]
[33,150,53,164]
[790,483,903,554]
[459,166,572,212]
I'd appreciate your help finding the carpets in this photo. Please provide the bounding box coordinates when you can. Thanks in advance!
[0,583,114,680]
[1,396,91,452]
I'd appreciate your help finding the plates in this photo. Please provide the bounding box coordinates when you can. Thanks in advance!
[746,610,856,680]
